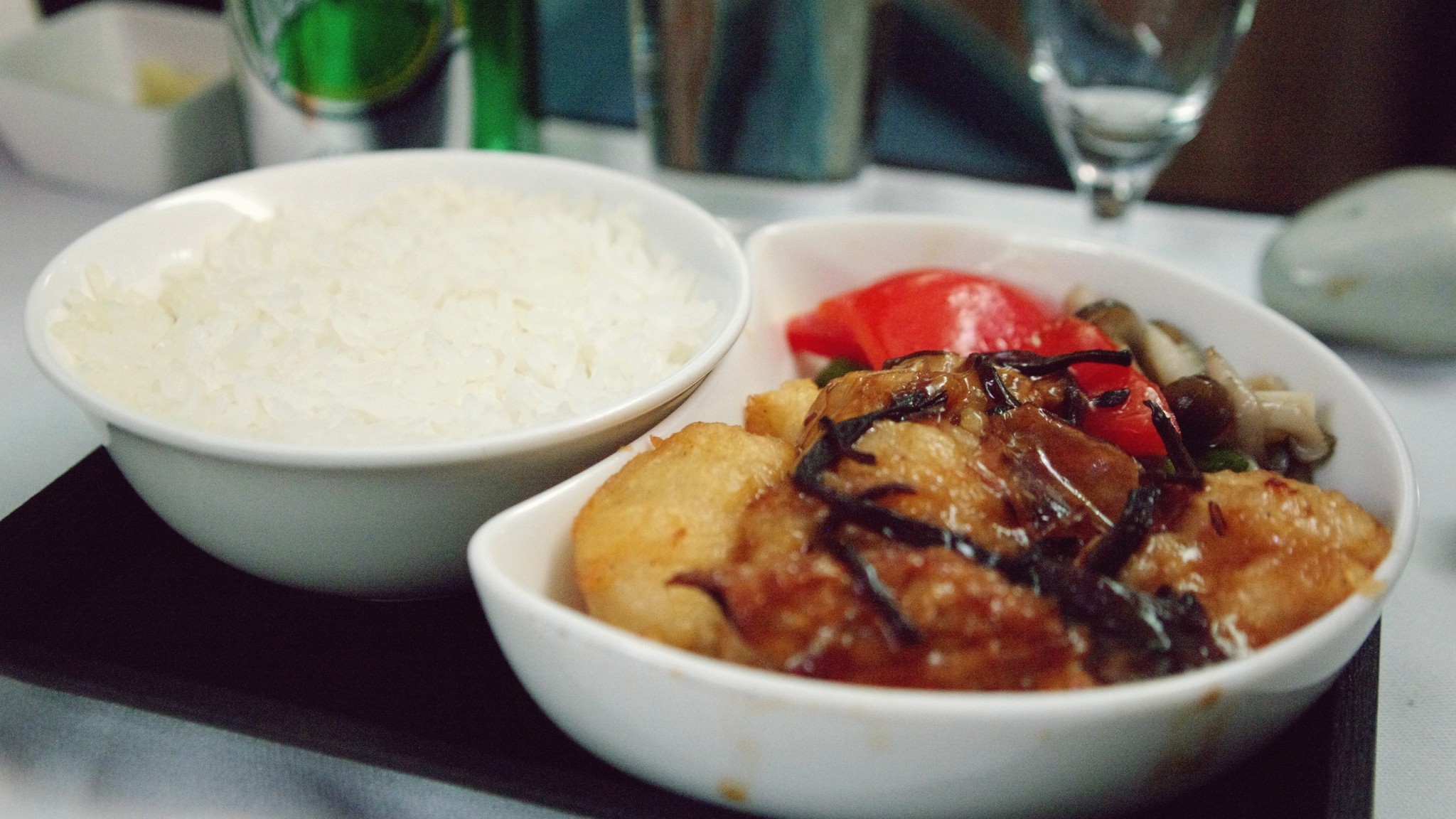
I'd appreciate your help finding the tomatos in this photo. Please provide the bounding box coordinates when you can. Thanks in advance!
[788,259,1181,457]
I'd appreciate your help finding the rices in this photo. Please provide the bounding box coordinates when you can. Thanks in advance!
[48,170,722,449]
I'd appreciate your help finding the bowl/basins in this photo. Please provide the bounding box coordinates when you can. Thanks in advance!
[26,150,750,596]
[0,3,244,197]
[472,212,1417,819]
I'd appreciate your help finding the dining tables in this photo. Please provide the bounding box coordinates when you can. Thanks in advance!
[7,90,1456,819]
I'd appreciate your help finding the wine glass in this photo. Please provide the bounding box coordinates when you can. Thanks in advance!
[1026,2,1260,232]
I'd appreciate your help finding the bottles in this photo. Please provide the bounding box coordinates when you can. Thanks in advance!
[226,4,540,169]
[623,2,884,178]
